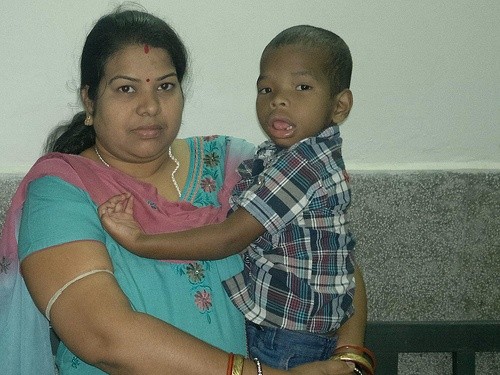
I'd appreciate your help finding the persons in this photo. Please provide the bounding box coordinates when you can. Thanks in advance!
[97,25,356,371]
[1,9,377,375]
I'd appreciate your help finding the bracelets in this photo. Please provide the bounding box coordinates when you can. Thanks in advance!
[250,358,263,375]
[232,354,244,375]
[329,344,376,375]
[227,353,234,375]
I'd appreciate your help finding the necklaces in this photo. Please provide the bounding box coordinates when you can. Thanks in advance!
[95,141,181,199]
